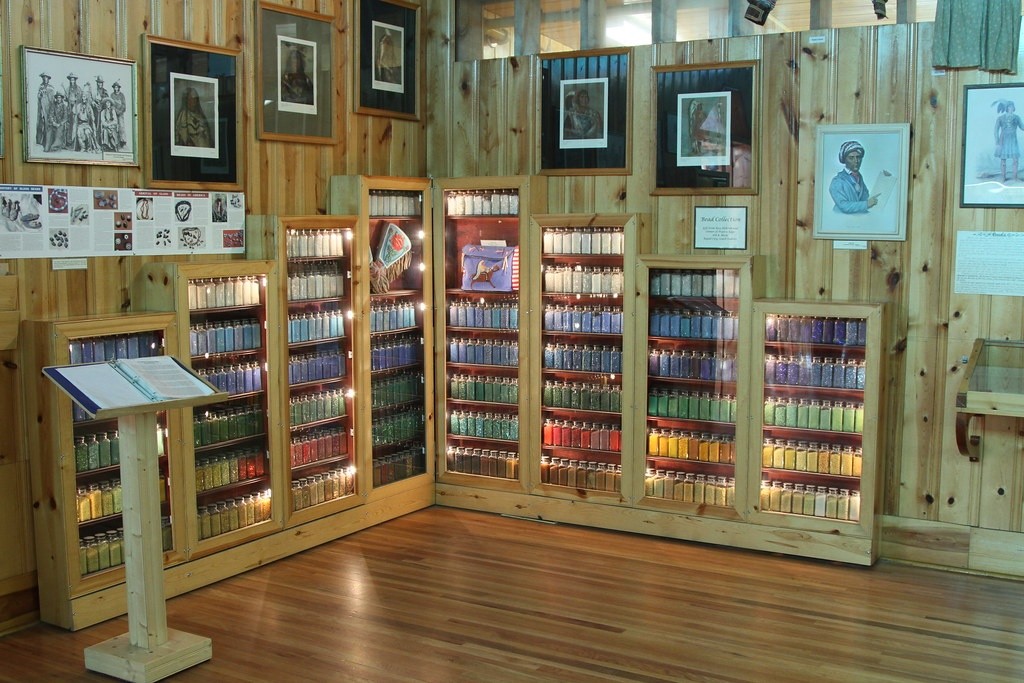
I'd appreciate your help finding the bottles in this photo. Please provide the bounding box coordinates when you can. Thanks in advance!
[643,266,739,507]
[447,189,518,215]
[447,297,521,479]
[759,314,866,522]
[369,298,424,489]
[539,227,624,492]
[368,189,423,216]
[285,226,353,512]
[74,274,265,577]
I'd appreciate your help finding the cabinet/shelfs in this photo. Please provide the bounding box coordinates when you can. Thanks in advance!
[433,177,888,566]
[21,174,436,632]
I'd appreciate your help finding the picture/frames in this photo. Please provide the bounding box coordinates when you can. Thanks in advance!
[142,33,244,192]
[960,83,1024,209]
[813,123,911,242]
[650,59,758,196]
[254,0,339,145]
[354,0,423,122]
[535,47,633,176]
[20,45,139,167]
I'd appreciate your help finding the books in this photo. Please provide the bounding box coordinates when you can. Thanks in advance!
[55,355,216,409]
